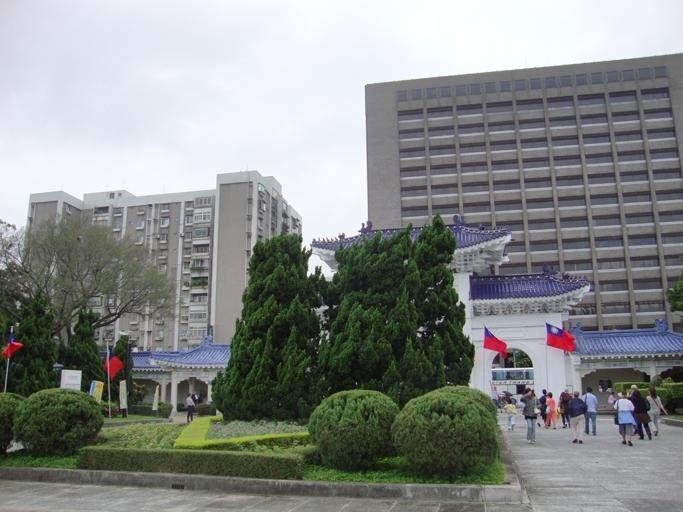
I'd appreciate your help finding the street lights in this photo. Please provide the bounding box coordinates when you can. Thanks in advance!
[2,322,21,397]
[51,358,64,389]
[104,337,113,417]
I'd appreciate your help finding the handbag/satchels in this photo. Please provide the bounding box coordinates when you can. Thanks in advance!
[546,406,550,414]
[614,414,619,425]
[534,408,541,414]
[560,407,565,414]
[644,399,651,412]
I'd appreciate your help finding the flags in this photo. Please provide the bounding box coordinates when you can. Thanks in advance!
[543,322,576,354]
[102,351,124,382]
[481,325,507,358]
[1,331,23,359]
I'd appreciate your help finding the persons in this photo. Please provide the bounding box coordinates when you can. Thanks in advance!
[502,384,669,446]
[185,392,205,424]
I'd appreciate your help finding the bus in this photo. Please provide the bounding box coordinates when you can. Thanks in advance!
[491,367,534,395]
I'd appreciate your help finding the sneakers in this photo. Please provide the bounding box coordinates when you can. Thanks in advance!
[619,429,658,447]
[507,422,597,444]
